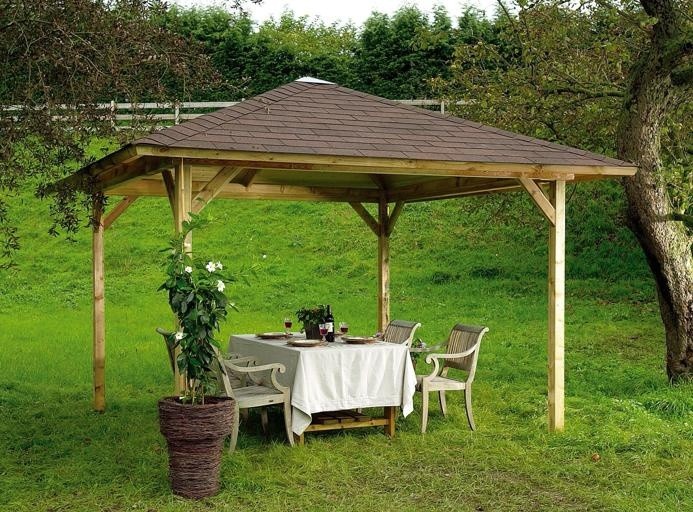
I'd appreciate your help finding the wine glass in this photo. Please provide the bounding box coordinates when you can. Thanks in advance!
[318,324,329,345]
[339,322,349,337]
[283,319,293,339]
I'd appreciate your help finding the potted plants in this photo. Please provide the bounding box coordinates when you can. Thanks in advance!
[295,305,326,340]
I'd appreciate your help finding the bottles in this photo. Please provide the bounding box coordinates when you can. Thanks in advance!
[325,304,335,343]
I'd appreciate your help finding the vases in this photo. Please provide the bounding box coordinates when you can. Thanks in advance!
[157,396,236,497]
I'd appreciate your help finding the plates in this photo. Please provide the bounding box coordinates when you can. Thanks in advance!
[342,337,378,344]
[286,339,323,346]
[257,332,290,340]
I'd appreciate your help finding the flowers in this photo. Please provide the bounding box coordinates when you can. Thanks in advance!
[157,195,261,405]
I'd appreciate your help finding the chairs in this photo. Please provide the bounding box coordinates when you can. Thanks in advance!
[156,328,296,449]
[356,320,422,414]
[408,323,490,435]
